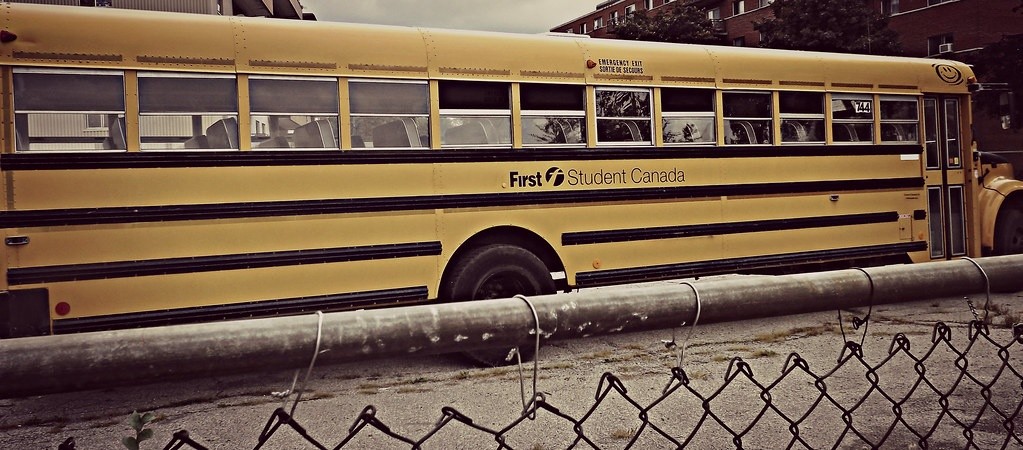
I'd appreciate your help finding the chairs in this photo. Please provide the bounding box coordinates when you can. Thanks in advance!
[16,118,906,150]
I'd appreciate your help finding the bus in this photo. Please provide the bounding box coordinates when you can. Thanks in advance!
[2,1,1023,374]
